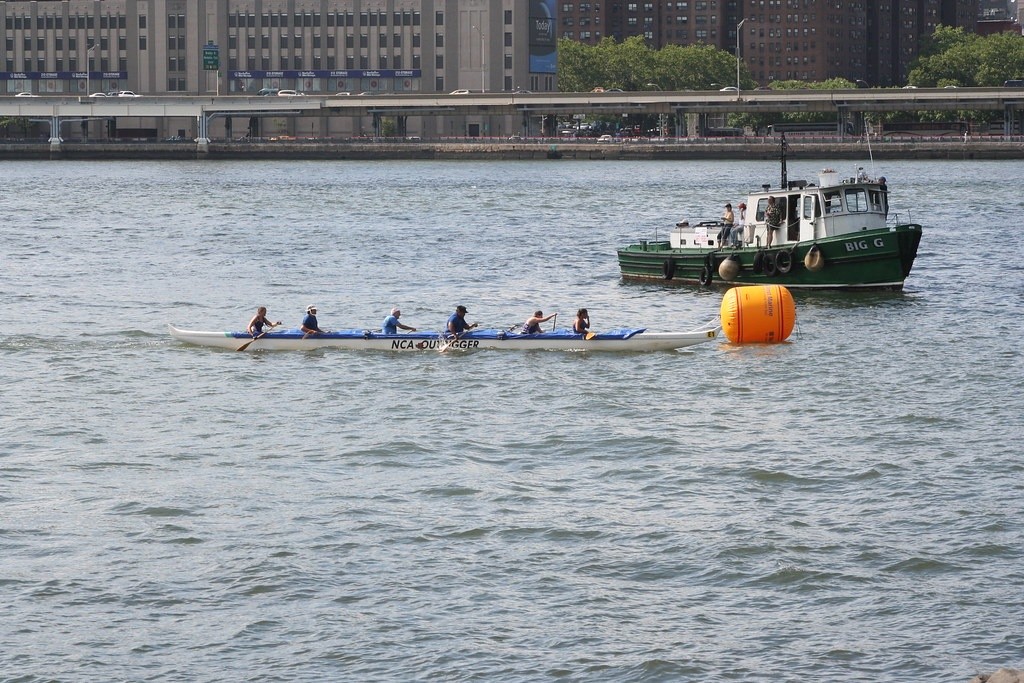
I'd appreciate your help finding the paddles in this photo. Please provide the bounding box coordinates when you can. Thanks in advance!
[439,324,476,352]
[235,322,278,352]
[302,330,332,340]
[552,313,557,331]
[404,330,412,334]
[585,332,595,340]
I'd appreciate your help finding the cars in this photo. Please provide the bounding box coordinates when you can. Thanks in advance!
[88,88,144,98]
[719,87,742,92]
[754,87,772,91]
[449,89,473,94]
[591,87,623,92]
[558,120,592,134]
[515,90,532,94]
[903,86,917,89]
[944,86,956,88]
[256,88,376,96]
[14,92,37,97]
[509,136,521,140]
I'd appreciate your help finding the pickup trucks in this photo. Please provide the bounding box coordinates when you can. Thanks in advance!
[597,134,616,144]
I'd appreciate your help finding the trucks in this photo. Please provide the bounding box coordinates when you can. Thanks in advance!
[271,136,295,141]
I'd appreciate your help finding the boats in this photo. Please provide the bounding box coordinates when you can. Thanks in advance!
[615,131,924,292]
[166,321,723,351]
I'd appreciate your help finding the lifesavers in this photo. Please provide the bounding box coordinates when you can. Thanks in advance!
[662,258,675,280]
[761,254,780,278]
[703,252,719,273]
[774,249,796,274]
[698,267,713,287]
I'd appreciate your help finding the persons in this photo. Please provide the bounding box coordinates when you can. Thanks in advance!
[248,307,282,339]
[382,307,416,336]
[717,204,734,251]
[445,306,477,339]
[573,309,590,334]
[300,304,321,334]
[875,177,889,221]
[730,203,746,248]
[520,311,557,335]
[764,196,779,249]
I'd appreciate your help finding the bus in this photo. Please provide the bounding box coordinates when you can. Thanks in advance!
[1005,80,1023,87]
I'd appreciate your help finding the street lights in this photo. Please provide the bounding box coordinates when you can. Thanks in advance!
[472,25,485,93]
[87,44,100,96]
[737,18,749,97]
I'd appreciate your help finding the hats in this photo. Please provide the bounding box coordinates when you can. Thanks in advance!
[391,307,400,315]
[725,204,732,207]
[457,305,469,313]
[304,304,319,311]
